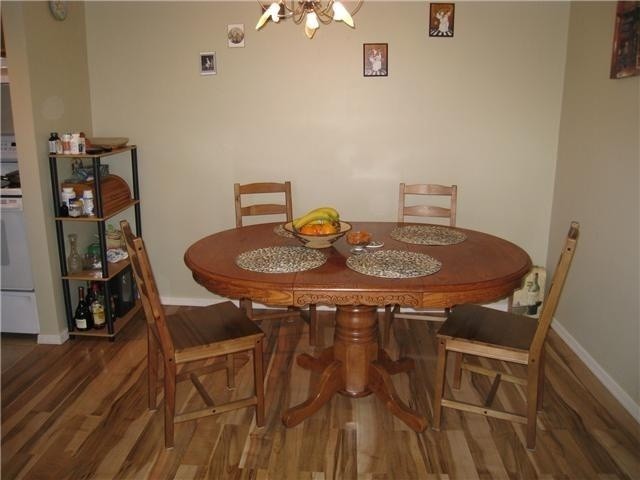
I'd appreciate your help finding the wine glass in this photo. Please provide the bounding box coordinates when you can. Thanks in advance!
[345,232,374,256]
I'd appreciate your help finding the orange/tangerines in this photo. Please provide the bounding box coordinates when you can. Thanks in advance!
[301,224,336,235]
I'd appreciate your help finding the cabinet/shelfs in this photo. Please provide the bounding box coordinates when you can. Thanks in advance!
[47,144,142,342]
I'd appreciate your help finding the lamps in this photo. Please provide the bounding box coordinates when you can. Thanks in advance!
[255,0,365,40]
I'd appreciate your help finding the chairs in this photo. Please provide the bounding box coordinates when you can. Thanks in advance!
[432,221,580,451]
[384,180,458,348]
[232,180,318,347]
[119,219,267,452]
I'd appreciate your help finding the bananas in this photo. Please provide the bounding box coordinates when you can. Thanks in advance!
[292,208,339,230]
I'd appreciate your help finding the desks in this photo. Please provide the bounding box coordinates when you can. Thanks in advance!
[183,220,534,434]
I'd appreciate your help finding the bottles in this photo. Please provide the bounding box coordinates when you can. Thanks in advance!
[526,272,541,315]
[60,187,94,217]
[67,233,101,275]
[75,281,118,331]
[48,132,91,155]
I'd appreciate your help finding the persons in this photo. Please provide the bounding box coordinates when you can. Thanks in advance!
[435,8,451,33]
[367,50,382,76]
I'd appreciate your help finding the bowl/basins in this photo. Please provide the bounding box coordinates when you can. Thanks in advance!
[283,220,352,248]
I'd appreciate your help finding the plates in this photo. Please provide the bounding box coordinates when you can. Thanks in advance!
[85,137,129,154]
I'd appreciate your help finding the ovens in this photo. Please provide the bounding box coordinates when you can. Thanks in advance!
[0,198,40,334]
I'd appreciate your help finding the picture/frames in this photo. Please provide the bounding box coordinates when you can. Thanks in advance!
[429,3,454,37]
[363,42,388,77]
[199,51,217,76]
[609,0,640,80]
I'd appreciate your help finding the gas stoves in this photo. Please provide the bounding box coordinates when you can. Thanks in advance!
[0,170,23,196]
